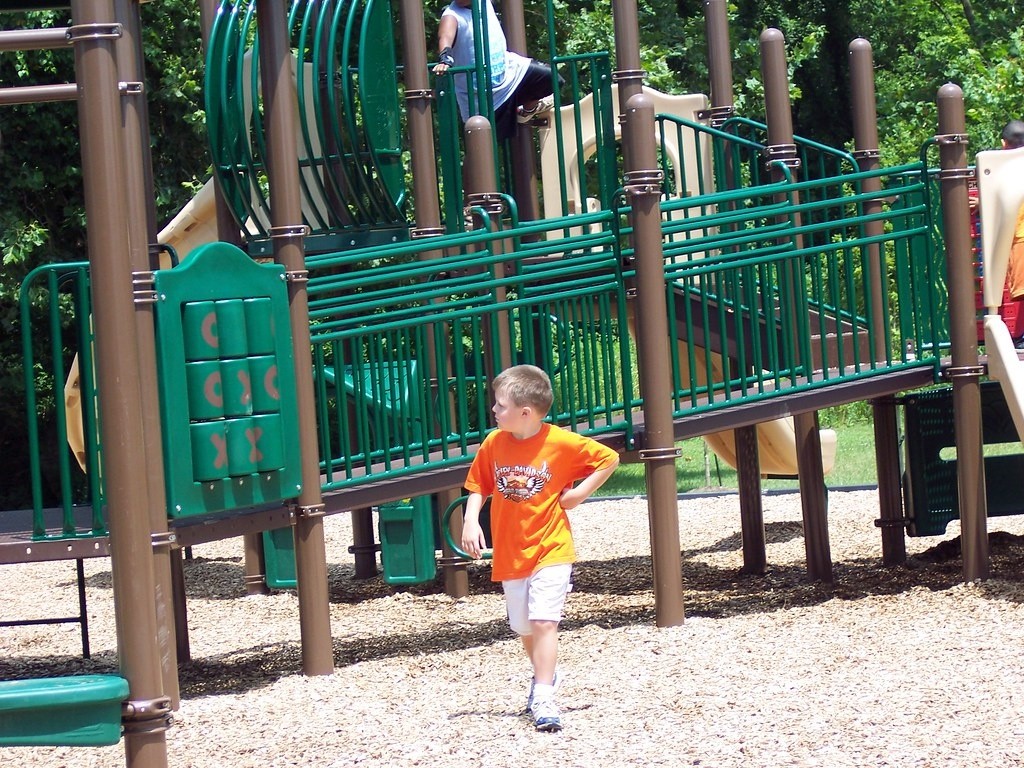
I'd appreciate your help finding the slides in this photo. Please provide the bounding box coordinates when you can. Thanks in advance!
[985,311,1024,449]
[627,301,837,475]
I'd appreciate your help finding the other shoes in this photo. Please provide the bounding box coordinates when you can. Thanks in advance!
[1015,333,1024,348]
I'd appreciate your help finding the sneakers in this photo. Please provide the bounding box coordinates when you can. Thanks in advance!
[517,93,554,123]
[456,207,475,226]
[530,695,564,730]
[526,672,562,713]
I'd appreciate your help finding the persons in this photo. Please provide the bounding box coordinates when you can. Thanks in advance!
[1001,119,1023,351]
[460,366,619,731]
[432,0,566,142]
